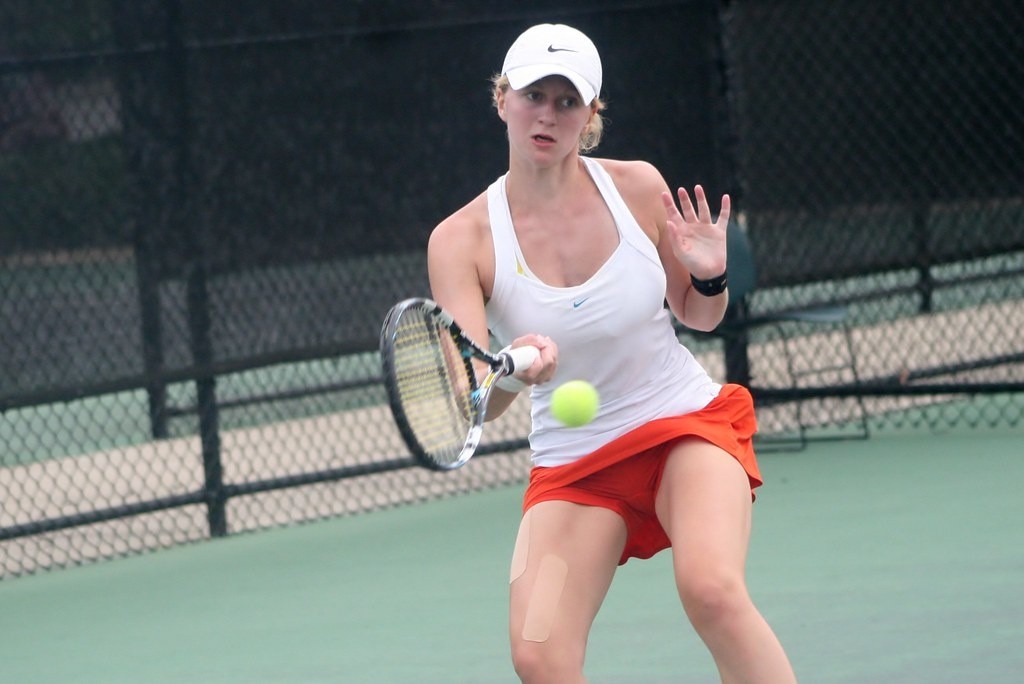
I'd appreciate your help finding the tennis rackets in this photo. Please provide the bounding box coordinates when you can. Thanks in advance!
[379,297,543,471]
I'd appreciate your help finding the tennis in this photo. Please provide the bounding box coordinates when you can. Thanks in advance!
[551,378,600,426]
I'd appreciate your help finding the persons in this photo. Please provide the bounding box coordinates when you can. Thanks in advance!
[428,22,798,684]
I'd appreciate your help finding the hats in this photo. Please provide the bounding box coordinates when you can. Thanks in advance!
[500,22,603,108]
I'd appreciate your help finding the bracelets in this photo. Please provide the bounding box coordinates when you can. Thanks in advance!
[690,272,728,298]
